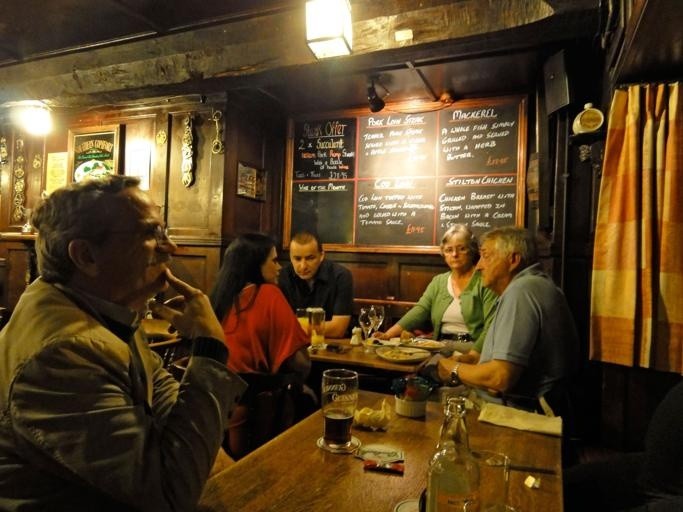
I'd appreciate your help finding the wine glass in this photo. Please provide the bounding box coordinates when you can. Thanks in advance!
[359,308,376,353]
[370,305,385,337]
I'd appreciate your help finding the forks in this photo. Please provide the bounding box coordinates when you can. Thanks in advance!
[396,350,440,355]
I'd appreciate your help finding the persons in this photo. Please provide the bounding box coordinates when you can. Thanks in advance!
[3,173,245,512]
[280,232,353,340]
[369,225,495,389]
[439,228,578,418]
[204,234,317,411]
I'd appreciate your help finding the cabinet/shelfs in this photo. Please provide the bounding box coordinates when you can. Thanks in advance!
[275,245,553,332]
[0,239,221,330]
[0,74,286,245]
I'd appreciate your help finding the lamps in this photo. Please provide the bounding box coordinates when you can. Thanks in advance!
[366,73,390,113]
[304,0,354,60]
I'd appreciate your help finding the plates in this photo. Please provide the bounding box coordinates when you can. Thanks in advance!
[376,347,431,363]
[362,339,400,349]
[402,338,443,349]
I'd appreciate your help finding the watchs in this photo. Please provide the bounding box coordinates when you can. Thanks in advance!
[452,363,460,384]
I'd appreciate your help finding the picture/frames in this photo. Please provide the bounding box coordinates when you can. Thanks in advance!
[236,160,268,202]
[67,124,120,191]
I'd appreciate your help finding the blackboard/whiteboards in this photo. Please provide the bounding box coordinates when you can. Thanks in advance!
[281,94,526,254]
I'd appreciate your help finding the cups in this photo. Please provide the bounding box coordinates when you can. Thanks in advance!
[477,449,511,512]
[296,308,309,336]
[321,369,359,448]
[311,311,325,348]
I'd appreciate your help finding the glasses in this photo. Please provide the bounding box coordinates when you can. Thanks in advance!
[103,221,172,244]
[443,244,473,257]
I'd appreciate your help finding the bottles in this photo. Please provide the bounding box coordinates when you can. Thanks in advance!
[424,398,481,511]
